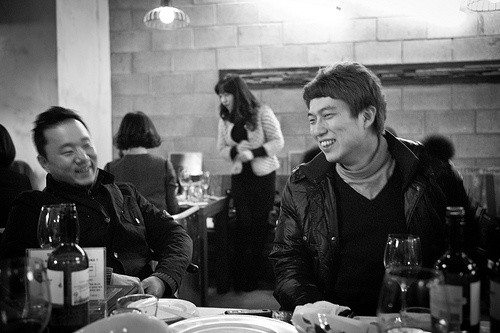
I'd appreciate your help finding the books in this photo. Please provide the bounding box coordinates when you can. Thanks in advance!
[88,284,138,324]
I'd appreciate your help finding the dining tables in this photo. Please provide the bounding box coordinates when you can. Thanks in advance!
[174,194,230,308]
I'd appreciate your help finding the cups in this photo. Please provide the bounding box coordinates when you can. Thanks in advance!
[116,293,158,316]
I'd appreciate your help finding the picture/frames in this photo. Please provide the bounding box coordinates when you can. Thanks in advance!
[168,152,203,175]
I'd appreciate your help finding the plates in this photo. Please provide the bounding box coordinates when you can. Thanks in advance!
[127,298,196,323]
[166,313,300,333]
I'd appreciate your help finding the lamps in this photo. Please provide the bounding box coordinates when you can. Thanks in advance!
[143,0,190,31]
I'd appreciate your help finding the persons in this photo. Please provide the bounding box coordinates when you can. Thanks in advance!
[104,109,180,214]
[1,123,32,230]
[214,75,285,291]
[1,106,193,299]
[267,60,500,320]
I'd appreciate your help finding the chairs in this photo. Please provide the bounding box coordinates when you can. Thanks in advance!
[166,206,202,309]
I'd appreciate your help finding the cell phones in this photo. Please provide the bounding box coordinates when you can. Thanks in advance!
[225,308,272,318]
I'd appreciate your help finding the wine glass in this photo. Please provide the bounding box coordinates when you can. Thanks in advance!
[377,233,450,333]
[0,257,52,333]
[199,171,210,202]
[38,202,79,249]
[178,166,191,202]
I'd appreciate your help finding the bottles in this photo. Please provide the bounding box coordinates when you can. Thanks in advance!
[486,233,500,333]
[428,206,481,333]
[47,202,90,333]
